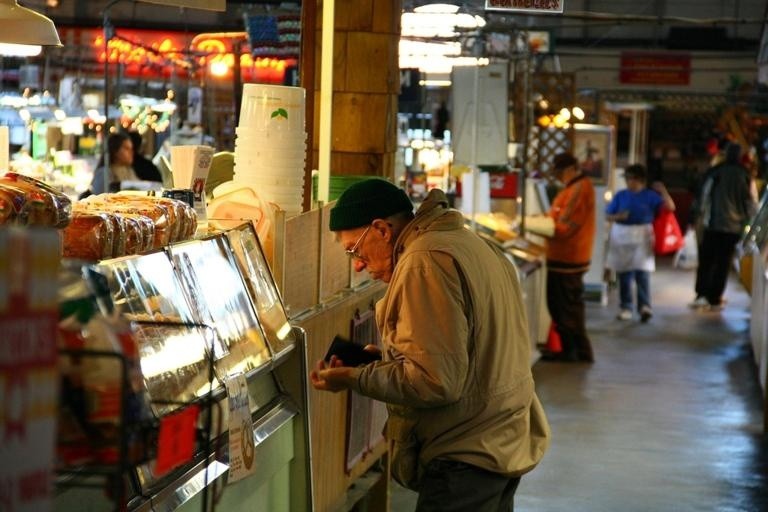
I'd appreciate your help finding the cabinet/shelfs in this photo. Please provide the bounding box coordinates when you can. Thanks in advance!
[214,325,314,512]
[55,317,218,511]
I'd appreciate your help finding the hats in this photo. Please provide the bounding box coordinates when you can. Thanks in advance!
[549,154,576,177]
[330,179,413,231]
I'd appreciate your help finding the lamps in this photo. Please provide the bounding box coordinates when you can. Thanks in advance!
[0,0,65,59]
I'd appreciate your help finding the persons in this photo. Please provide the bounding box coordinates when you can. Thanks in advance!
[90,132,138,195]
[515,152,596,366]
[689,143,756,312]
[605,163,676,322]
[309,180,553,511]
[128,130,162,183]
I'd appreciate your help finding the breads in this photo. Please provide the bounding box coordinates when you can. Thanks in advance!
[0,171,199,257]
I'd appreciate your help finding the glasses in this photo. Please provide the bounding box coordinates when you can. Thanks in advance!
[345,227,369,257]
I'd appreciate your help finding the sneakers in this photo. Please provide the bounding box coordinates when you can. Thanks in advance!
[641,305,652,321]
[618,309,633,319]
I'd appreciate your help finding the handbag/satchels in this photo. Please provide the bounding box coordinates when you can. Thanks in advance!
[653,205,683,256]
